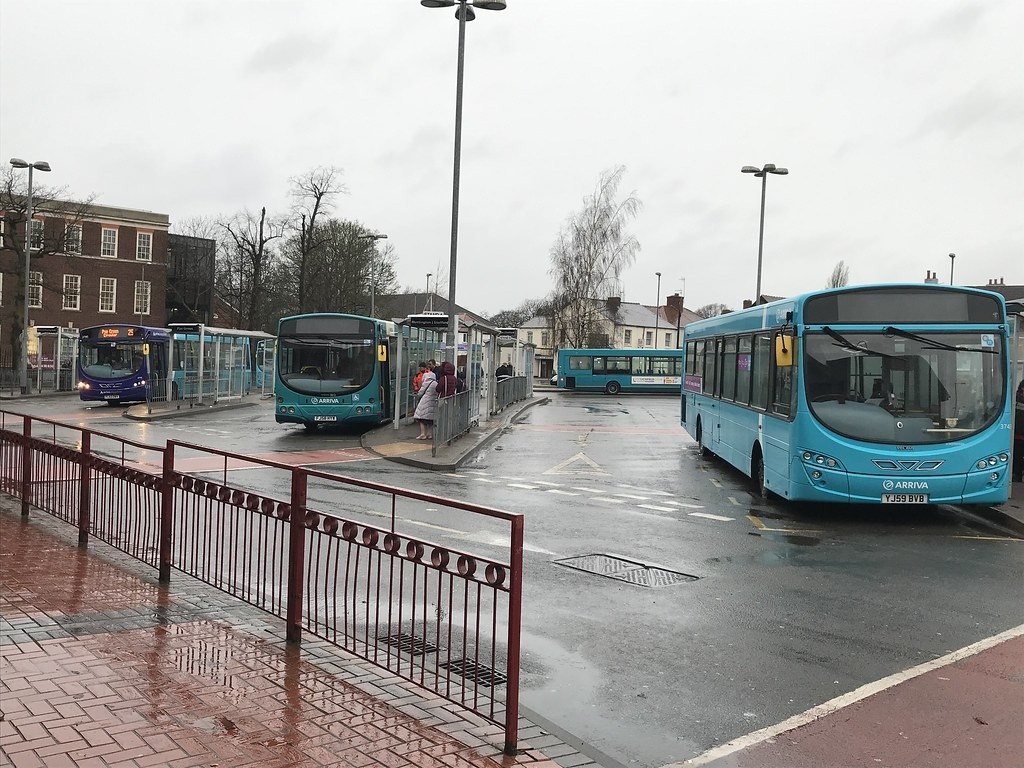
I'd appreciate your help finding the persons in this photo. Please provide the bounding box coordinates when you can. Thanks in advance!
[496,361,516,381]
[413,359,465,440]
[337,351,350,371]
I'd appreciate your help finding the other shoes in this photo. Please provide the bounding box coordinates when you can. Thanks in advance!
[416,435,426,440]
[426,434,432,438]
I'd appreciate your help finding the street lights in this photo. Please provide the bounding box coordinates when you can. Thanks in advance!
[655,272,662,349]
[10,158,51,394]
[357,233,388,317]
[426,274,433,315]
[741,164,789,306]
[950,253,956,286]
[420,0,507,361]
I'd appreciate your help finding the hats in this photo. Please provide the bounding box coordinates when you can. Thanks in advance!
[419,362,426,367]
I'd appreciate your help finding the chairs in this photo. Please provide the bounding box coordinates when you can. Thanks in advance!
[663,370,666,374]
[612,363,618,371]
[648,370,653,374]
[640,369,642,373]
[335,357,361,378]
[658,370,661,374]
[576,362,580,369]
[637,369,639,374]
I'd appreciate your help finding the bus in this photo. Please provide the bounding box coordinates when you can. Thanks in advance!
[275,313,482,431]
[556,348,683,394]
[682,284,1013,506]
[78,324,276,406]
